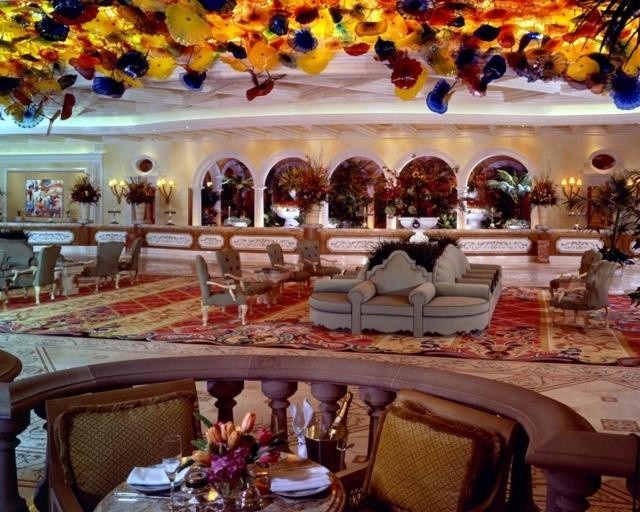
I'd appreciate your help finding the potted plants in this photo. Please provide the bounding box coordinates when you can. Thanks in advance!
[71,175,97,219]
[201,154,556,229]
[582,169,640,295]
[127,176,154,222]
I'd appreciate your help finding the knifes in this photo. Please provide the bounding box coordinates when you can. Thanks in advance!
[113,488,180,500]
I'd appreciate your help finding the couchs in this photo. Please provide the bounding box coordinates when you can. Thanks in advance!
[307,243,503,338]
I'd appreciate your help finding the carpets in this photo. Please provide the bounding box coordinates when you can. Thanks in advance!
[0,277,640,367]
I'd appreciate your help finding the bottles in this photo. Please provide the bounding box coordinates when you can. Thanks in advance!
[326,391,354,438]
[255,470,269,495]
[186,470,209,511]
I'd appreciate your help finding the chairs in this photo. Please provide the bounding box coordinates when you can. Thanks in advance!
[194,254,252,327]
[353,387,522,512]
[295,241,343,289]
[549,249,603,318]
[0,238,142,303]
[35,378,203,512]
[266,243,311,299]
[215,247,276,309]
[547,260,616,334]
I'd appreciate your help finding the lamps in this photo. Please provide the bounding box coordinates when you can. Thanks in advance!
[562,177,582,202]
[157,178,176,205]
[109,179,126,203]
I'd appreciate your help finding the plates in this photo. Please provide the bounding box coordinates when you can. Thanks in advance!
[129,480,181,492]
[270,471,331,498]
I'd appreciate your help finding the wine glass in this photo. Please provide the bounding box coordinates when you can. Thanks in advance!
[160,435,183,510]
[258,413,279,482]
